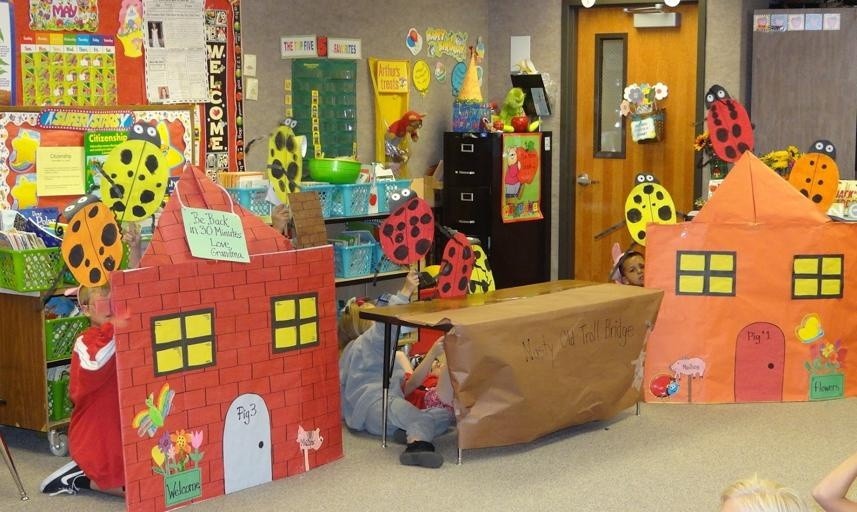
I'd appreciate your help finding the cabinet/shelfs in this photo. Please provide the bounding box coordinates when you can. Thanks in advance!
[0,286,89,434]
[322,209,418,287]
[441,130,553,292]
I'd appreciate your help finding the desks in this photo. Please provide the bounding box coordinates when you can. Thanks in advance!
[358,278,665,465]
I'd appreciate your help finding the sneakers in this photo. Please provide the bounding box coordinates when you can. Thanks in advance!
[40,460,87,496]
[394,428,443,468]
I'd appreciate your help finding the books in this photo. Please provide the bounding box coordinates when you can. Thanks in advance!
[0,213,63,288]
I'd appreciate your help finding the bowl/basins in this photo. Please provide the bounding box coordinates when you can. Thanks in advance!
[308,158,362,184]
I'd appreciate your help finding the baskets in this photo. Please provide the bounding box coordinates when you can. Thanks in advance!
[291,58,357,161]
[226,180,413,278]
[0,240,129,421]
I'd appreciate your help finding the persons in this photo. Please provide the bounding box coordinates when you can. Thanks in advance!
[40,283,127,496]
[720,452,857,512]
[613,250,645,287]
[339,270,454,467]
